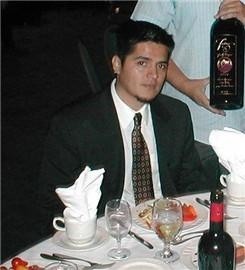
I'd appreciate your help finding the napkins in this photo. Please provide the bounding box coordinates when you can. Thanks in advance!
[54,166,106,220]
[208,127,245,185]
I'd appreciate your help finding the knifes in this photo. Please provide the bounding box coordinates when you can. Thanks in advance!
[112,218,153,249]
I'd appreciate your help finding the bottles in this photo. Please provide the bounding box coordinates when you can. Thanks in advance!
[210,17,245,110]
[198,189,234,270]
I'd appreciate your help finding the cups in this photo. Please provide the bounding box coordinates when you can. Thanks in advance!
[53,209,97,244]
[220,173,245,202]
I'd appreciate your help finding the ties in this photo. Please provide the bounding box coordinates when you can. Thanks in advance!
[132,112,155,207]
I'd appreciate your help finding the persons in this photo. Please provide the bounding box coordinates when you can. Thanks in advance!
[130,0,245,145]
[38,22,208,217]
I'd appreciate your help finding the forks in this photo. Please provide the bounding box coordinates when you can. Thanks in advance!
[40,254,116,270]
[196,198,239,219]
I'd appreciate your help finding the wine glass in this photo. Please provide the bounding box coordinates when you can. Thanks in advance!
[105,199,132,260]
[151,198,183,263]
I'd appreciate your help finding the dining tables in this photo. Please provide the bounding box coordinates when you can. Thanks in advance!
[0,189,245,270]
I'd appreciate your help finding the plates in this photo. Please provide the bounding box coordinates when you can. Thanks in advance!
[132,199,208,233]
[221,189,245,206]
[54,226,108,251]
